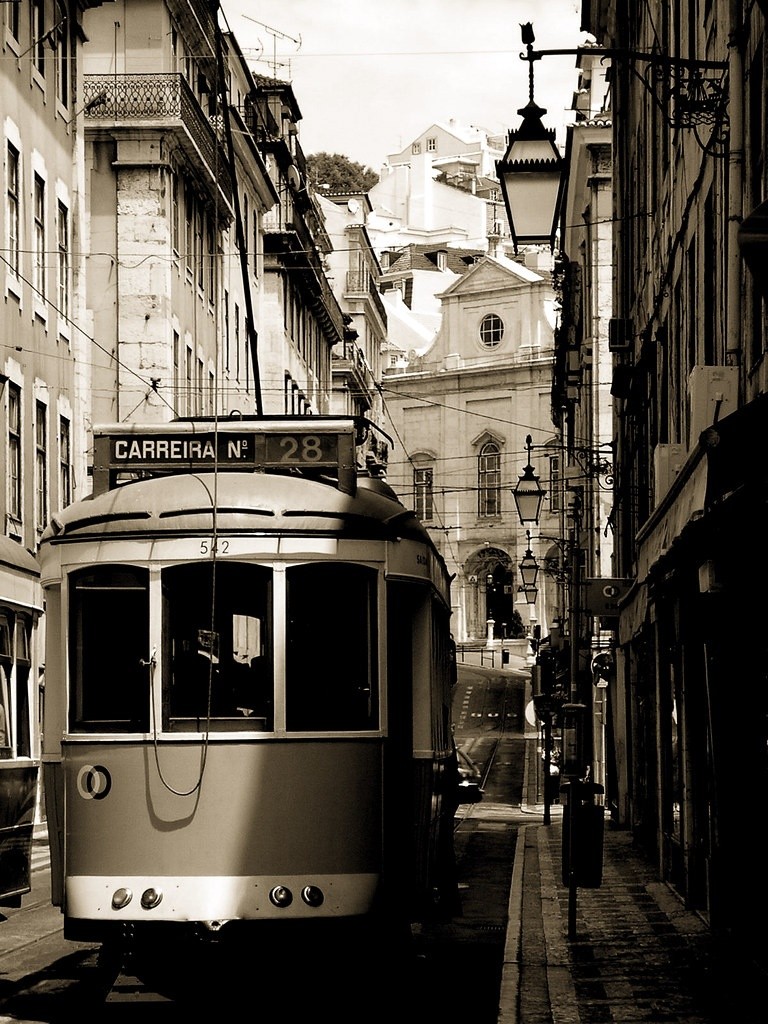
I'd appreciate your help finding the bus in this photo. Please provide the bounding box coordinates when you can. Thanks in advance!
[34,409,458,972]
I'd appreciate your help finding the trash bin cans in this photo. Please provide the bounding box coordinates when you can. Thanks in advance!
[503,649,510,664]
[562,779,606,888]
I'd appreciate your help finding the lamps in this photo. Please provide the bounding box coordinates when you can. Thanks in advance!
[510,432,620,605]
[494,21,732,257]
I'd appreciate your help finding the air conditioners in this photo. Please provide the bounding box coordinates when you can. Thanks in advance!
[650,442,684,516]
[683,366,739,456]
